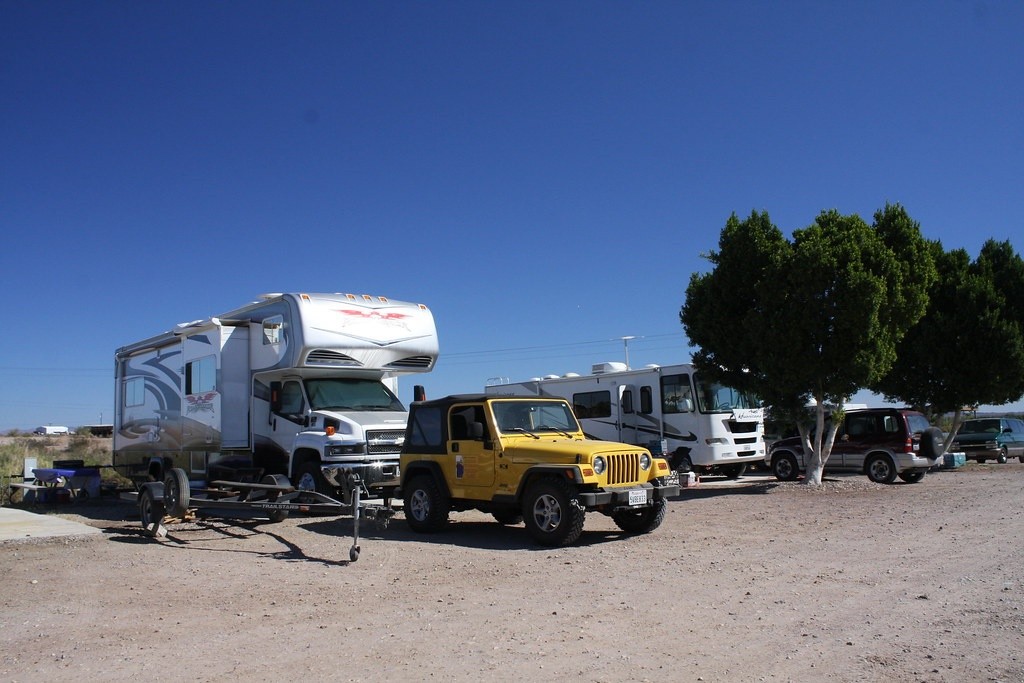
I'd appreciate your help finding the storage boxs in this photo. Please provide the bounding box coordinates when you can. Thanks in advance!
[943,452,966,469]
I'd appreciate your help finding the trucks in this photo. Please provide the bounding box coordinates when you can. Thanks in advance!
[108,291,440,504]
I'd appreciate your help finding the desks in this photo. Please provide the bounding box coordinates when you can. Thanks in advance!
[32,468,100,504]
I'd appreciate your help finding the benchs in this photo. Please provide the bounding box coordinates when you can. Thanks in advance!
[101,483,119,498]
[9,482,53,507]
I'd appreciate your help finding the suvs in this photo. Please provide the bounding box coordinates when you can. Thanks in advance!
[397,392,680,548]
[950,416,1024,465]
[764,405,945,485]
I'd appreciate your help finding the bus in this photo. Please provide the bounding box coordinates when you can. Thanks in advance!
[484,362,767,479]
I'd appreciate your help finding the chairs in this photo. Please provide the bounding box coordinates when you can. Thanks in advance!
[848,418,898,434]
[665,388,709,411]
[469,421,483,441]
